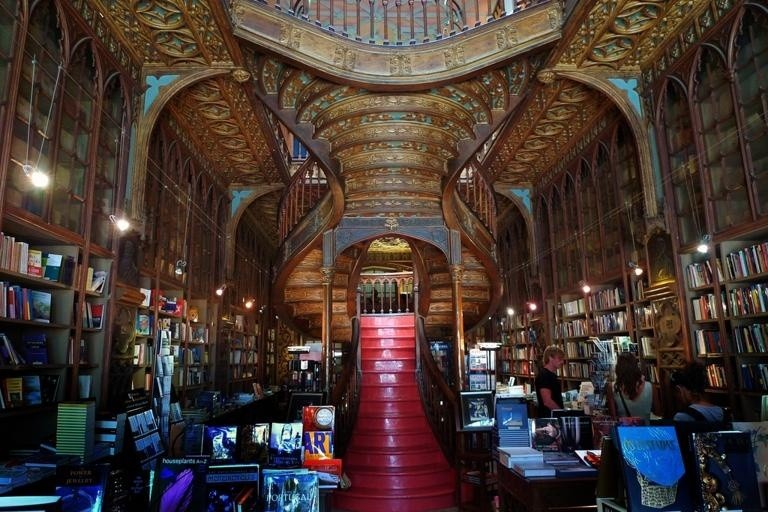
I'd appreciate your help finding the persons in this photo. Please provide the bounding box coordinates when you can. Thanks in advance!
[606,352,662,427]
[534,347,565,416]
[672,362,733,422]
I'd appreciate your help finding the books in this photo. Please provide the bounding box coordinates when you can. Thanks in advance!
[688,431,762,512]
[611,426,697,512]
[595,436,625,499]
[731,421,768,483]
[460,391,597,478]
[0,229,344,512]
[427,239,768,390]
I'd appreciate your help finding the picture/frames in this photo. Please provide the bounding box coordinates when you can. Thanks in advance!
[284,390,327,424]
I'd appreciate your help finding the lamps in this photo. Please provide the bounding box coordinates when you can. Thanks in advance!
[666,29,715,254]
[15,48,72,193]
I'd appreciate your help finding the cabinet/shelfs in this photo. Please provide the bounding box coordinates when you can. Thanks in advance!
[0,208,277,450]
[484,224,768,434]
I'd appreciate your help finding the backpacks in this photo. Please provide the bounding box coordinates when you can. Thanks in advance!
[679,403,735,431]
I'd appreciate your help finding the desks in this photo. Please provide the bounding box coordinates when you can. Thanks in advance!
[495,455,600,511]
[459,447,495,511]
[1,388,281,512]
